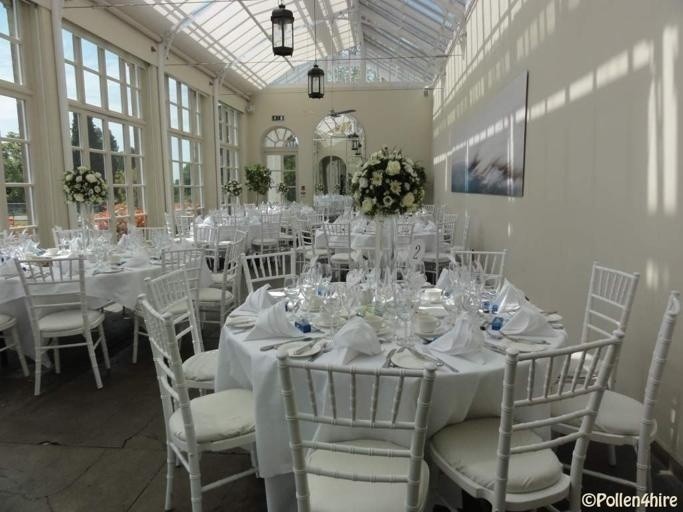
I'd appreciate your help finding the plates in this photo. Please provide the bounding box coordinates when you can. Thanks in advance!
[501,339,548,352]
[226,315,256,330]
[277,339,319,359]
[390,350,439,370]
[541,312,561,321]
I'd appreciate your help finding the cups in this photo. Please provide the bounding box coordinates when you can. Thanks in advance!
[417,315,441,333]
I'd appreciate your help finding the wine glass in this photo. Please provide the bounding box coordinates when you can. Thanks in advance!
[300,248,501,346]
[283,274,300,321]
[9,224,163,270]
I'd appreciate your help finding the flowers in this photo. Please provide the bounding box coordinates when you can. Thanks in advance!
[60,166,109,207]
[220,144,428,218]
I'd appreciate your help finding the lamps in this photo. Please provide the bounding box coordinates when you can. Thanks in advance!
[270,0,362,156]
